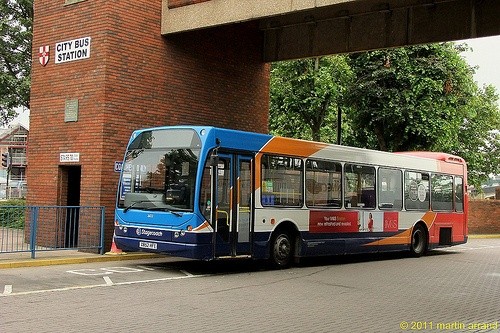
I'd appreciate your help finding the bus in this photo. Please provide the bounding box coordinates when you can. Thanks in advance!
[112,125,469,270]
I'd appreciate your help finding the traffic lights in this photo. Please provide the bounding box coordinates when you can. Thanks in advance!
[2,152,8,168]
